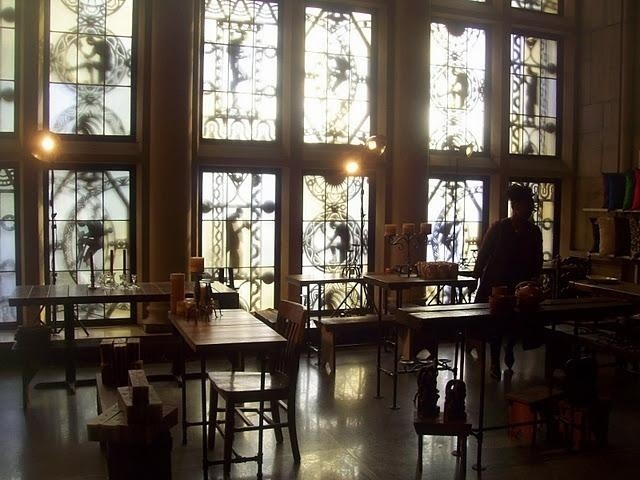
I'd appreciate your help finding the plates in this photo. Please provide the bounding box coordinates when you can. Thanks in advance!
[586,273,618,283]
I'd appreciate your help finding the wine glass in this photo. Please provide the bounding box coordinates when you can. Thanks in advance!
[119,274,137,290]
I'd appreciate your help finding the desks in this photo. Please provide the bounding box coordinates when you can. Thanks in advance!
[394,292,640,472]
[167,308,289,479]
[9,280,238,395]
[286,274,478,399]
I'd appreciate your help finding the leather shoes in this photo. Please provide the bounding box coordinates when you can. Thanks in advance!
[504,347,515,369]
[489,362,501,384]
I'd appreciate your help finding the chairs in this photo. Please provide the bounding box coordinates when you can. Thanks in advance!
[208,298,309,472]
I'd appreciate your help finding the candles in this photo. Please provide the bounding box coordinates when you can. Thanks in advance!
[420,223,431,234]
[402,223,415,234]
[385,224,396,235]
[189,257,204,273]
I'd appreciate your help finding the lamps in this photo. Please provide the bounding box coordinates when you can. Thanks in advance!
[428,144,472,305]
[29,129,89,335]
[331,154,379,318]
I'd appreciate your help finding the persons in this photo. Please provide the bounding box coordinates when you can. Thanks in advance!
[472,183,544,381]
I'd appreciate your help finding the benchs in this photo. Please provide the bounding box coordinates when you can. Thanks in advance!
[95,370,173,479]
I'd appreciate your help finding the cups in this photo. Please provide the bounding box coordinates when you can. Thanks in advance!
[200,278,211,288]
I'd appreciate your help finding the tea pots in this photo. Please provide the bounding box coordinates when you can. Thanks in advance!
[98,271,116,290]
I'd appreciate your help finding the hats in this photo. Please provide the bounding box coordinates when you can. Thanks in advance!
[509,184,535,198]
